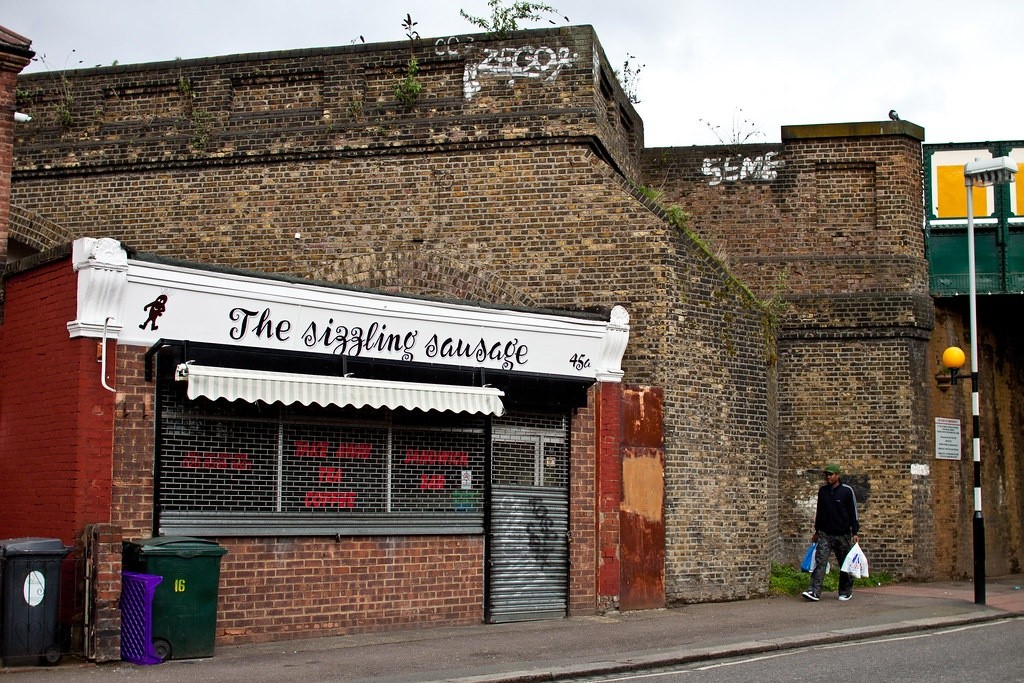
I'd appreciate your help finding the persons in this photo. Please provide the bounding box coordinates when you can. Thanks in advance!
[801,464,859,601]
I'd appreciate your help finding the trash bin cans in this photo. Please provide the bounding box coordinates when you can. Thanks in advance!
[1,535,76,665]
[123,536,230,657]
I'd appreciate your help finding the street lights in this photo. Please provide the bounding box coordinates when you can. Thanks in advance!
[943,152,1019,607]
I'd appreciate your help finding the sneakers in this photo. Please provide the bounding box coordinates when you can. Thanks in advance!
[802,590,820,600]
[838,595,853,601]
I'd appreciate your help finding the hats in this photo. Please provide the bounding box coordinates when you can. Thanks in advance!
[822,463,840,474]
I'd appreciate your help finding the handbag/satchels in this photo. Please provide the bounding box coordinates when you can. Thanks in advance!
[840,541,869,579]
[801,542,830,574]
